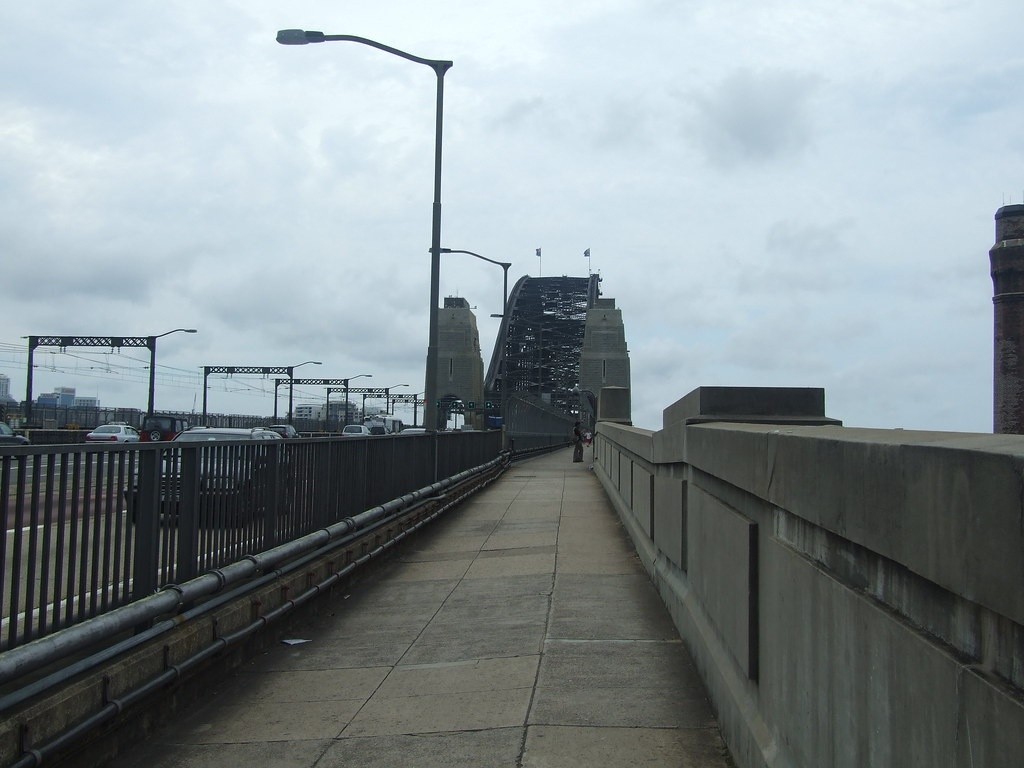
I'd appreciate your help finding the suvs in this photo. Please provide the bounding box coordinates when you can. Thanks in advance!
[124,425,289,523]
[140,415,189,441]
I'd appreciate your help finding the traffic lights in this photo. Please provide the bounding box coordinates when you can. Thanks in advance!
[437,402,439,406]
[469,402,474,408]
[486,402,492,409]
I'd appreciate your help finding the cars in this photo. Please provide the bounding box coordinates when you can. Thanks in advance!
[268,425,301,439]
[85,425,141,453]
[370,427,385,435]
[341,425,372,436]
[0,422,31,459]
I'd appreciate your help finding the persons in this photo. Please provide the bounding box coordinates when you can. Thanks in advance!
[573,422,584,462]
[583,430,593,447]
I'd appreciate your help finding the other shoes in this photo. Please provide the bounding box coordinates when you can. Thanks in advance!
[573,460,584,462]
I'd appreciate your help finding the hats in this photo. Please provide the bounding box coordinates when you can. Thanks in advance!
[575,422,581,426]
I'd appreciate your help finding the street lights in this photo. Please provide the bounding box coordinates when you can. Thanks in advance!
[490,314,542,398]
[386,384,409,418]
[429,246,512,417]
[276,29,453,432]
[286,360,322,426]
[344,374,372,424]
[146,328,198,431]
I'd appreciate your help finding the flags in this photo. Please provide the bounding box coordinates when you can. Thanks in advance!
[583,248,590,257]
[536,249,541,256]
